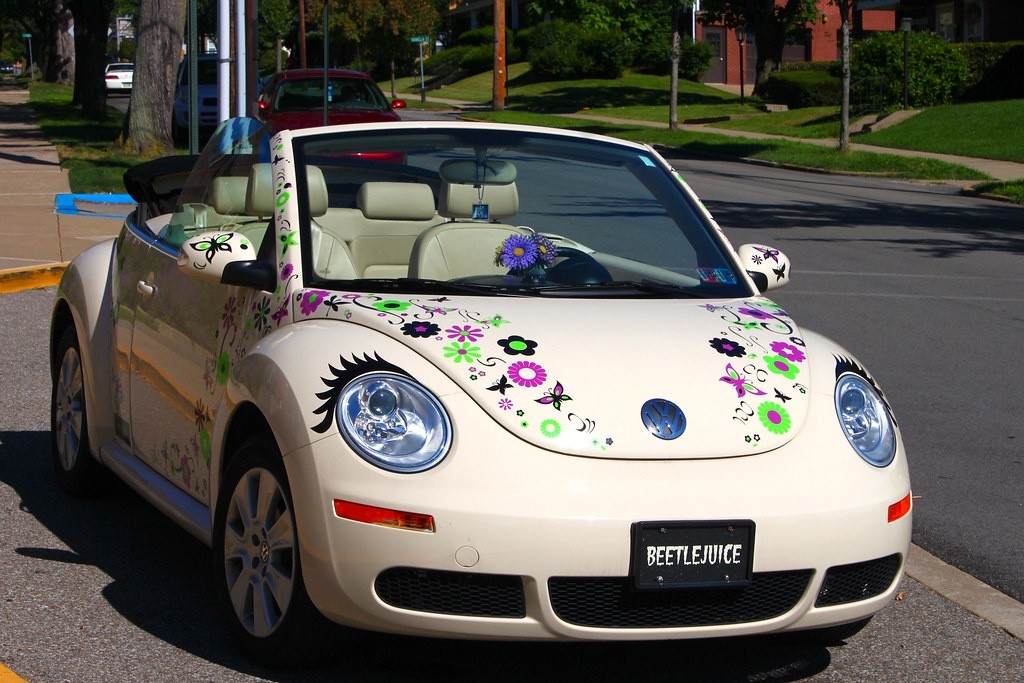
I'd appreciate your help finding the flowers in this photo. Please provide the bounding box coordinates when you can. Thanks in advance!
[493,231,557,275]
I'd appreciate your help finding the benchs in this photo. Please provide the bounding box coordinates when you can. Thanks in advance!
[171,176,449,279]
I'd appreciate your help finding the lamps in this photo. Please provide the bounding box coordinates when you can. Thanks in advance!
[823,14,828,23]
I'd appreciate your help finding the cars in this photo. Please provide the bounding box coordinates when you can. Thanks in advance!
[106,63,134,95]
[48,117,914,656]
[0,64,14,74]
[257,69,407,167]
[174,54,219,139]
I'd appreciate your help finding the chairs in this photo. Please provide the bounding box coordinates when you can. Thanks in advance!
[408,179,530,282]
[235,163,360,281]
